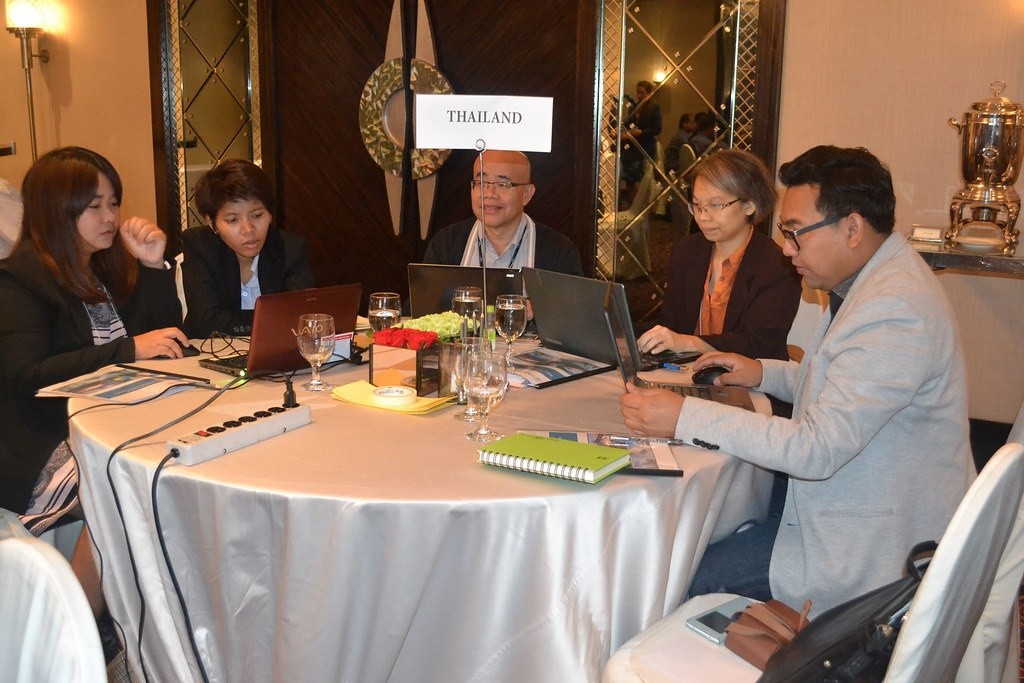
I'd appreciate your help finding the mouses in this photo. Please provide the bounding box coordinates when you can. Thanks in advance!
[691,366,731,386]
[152,338,200,359]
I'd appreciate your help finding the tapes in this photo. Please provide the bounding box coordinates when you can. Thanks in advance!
[371,386,417,406]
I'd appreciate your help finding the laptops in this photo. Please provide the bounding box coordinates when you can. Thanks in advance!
[602,280,760,411]
[200,282,364,377]
[523,268,704,373]
[408,262,523,338]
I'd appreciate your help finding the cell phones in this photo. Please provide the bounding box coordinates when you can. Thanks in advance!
[686,597,754,644]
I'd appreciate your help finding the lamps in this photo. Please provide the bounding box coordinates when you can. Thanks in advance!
[7,0,48,167]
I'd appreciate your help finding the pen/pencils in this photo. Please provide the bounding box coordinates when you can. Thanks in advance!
[609,434,682,447]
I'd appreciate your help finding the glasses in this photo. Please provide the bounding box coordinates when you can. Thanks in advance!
[776,209,866,251]
[470,180,530,194]
[687,197,742,217]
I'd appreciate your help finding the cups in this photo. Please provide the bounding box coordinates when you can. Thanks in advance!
[441,335,463,397]
[368,292,402,332]
[451,286,484,320]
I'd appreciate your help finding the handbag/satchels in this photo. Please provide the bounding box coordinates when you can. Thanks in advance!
[756,540,938,683]
[723,598,811,672]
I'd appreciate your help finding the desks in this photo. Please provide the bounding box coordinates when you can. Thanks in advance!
[66,330,773,682]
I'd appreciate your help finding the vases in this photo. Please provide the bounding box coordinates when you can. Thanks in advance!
[368,331,481,396]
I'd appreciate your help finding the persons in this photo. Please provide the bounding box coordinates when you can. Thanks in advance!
[178,157,317,337]
[636,148,803,362]
[665,112,713,173]
[422,149,584,321]
[621,145,978,622]
[622,80,663,208]
[0,146,189,624]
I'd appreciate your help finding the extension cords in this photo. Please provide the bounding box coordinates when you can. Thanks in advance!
[167,402,311,466]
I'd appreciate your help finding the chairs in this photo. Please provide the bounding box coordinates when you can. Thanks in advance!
[601,403,1024,683]
[620,138,696,283]
[0,536,109,683]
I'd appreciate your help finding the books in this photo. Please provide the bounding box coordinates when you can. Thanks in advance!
[475,431,631,484]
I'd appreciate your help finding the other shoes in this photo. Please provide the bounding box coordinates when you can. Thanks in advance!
[98,618,118,659]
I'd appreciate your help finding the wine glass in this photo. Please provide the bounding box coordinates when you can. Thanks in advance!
[298,314,335,391]
[464,351,507,442]
[494,295,527,371]
[454,337,492,422]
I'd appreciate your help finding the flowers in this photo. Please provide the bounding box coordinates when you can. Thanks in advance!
[375,309,471,346]
